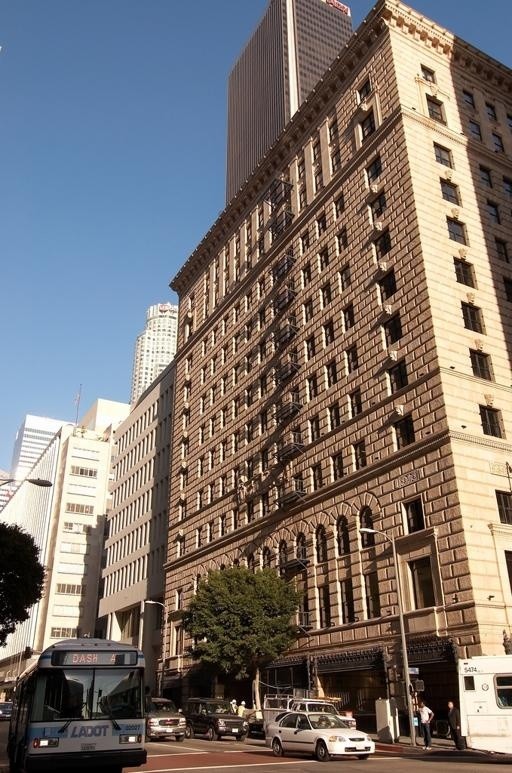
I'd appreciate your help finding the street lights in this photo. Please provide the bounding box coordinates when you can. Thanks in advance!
[0,475,53,494]
[357,525,417,746]
[53,629,73,638]
[144,600,167,697]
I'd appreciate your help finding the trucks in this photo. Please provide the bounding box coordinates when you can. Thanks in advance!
[457,653,512,766]
[260,690,358,734]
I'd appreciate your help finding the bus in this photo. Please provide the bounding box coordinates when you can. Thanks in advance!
[7,635,149,771]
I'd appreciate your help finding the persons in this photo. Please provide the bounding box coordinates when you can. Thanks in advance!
[231,699,238,715]
[318,717,335,728]
[448,700,464,751]
[418,701,434,750]
[216,705,226,714]
[238,701,246,717]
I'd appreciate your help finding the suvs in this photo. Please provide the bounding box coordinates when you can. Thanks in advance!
[180,697,250,744]
[0,702,13,721]
[146,695,188,743]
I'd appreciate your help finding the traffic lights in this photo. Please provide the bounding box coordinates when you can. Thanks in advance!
[23,646,31,658]
[413,678,425,692]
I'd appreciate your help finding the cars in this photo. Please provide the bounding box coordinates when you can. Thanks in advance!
[263,711,377,760]
